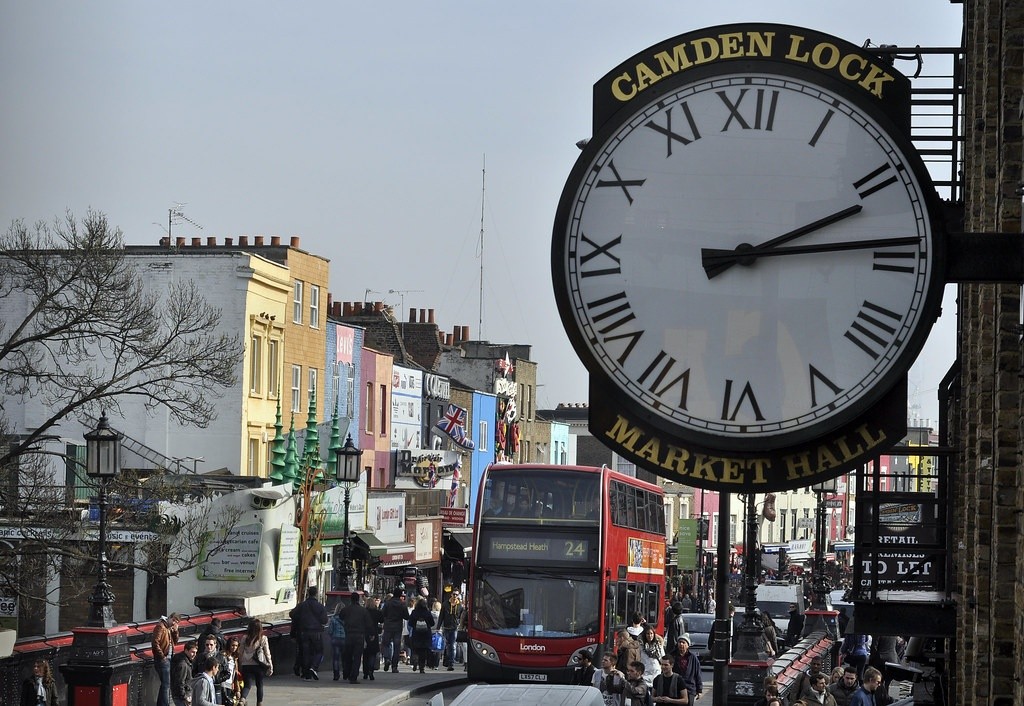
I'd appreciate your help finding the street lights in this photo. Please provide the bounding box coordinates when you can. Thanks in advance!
[809,474,838,612]
[334,432,366,591]
[66,410,134,664]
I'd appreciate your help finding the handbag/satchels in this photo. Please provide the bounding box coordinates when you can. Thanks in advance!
[456,628,468,642]
[214,665,231,684]
[253,636,268,665]
[431,631,445,653]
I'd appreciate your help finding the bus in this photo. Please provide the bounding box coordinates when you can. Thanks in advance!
[453,460,667,687]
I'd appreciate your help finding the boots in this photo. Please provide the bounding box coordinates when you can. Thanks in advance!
[333,670,339,680]
[343,670,348,679]
[448,661,454,670]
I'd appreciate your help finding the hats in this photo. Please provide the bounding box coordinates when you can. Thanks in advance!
[676,632,690,645]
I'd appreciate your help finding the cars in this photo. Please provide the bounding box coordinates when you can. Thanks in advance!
[663,613,715,666]
[734,607,761,633]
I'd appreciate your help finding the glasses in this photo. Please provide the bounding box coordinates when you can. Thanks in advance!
[790,606,795,608]
[577,657,583,660]
[205,644,215,646]
[452,593,458,596]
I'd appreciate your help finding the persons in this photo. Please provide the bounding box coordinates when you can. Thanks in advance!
[21,659,58,706]
[328,593,469,683]
[587,498,599,518]
[664,570,778,632]
[152,612,273,706]
[574,602,703,706]
[757,635,905,706]
[482,496,551,518]
[288,587,328,680]
[706,604,804,659]
[793,565,852,609]
[474,612,481,630]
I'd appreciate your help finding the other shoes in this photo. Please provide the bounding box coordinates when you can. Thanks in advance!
[369,671,374,680]
[384,661,391,671]
[238,701,244,706]
[304,678,311,681]
[420,669,425,673]
[413,660,418,671]
[293,666,300,676]
[392,667,399,673]
[363,674,368,679]
[349,680,359,684]
[309,668,318,680]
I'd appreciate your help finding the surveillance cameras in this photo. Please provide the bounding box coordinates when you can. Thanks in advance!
[903,636,925,655]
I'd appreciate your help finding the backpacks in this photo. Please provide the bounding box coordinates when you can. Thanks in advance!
[415,614,429,633]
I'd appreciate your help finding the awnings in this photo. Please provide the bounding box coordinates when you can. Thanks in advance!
[445,534,471,561]
[352,535,387,558]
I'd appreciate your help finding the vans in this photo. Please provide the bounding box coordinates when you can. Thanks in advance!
[753,579,805,638]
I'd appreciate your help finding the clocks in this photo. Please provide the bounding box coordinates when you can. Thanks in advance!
[550,56,951,453]
